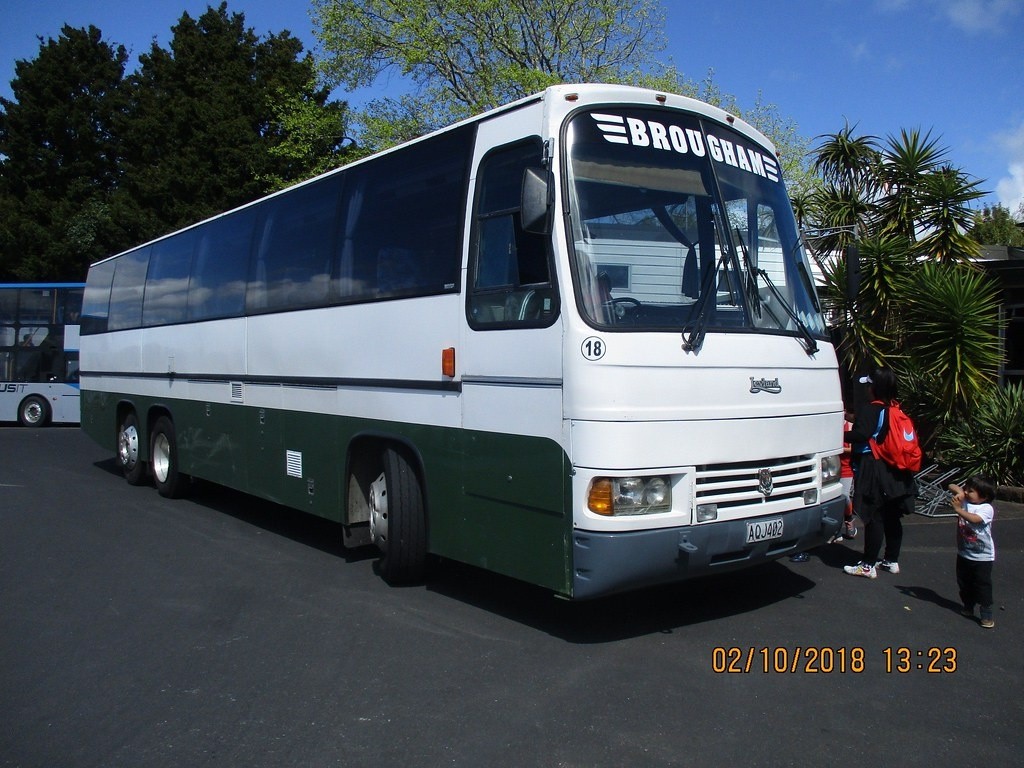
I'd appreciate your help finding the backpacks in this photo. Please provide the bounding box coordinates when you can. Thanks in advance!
[868,399,921,472]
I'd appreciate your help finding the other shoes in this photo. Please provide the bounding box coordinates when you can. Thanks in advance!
[960,606,975,616]
[980,607,994,627]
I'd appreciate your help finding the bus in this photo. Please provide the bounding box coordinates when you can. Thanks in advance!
[0,282,89,429]
[78,83,854,605]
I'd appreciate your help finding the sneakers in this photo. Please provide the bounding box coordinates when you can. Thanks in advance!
[845,518,858,538]
[844,561,877,578]
[875,559,899,574]
[826,531,843,543]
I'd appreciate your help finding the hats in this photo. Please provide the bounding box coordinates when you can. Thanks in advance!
[859,375,873,383]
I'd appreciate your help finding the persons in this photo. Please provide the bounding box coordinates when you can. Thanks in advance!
[844,367,920,580]
[791,550,811,563]
[948,474,996,628]
[19,334,35,347]
[826,405,858,544]
